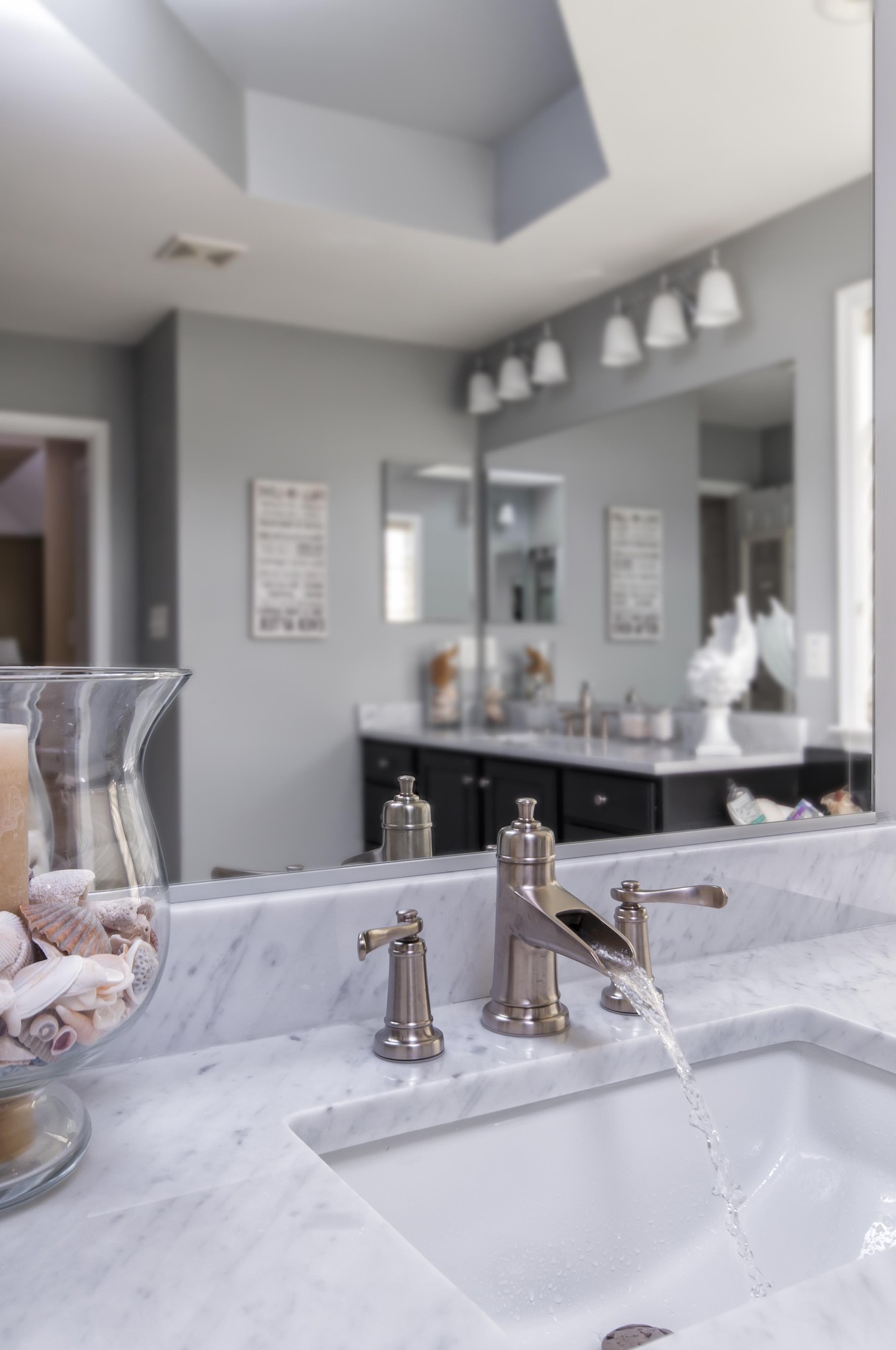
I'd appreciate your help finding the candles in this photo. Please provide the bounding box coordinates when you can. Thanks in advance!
[2,723,40,1166]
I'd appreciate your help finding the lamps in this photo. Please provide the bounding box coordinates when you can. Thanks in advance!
[466,323,566,415]
[598,240,743,367]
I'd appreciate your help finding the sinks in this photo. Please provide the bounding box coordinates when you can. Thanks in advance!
[290,1001,896,1348]
[494,726,641,758]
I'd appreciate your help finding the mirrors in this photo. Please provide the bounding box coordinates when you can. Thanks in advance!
[1,0,874,895]
[477,355,798,709]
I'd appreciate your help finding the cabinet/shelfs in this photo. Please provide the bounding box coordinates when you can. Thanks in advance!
[416,746,557,857]
[359,736,417,857]
[558,763,803,847]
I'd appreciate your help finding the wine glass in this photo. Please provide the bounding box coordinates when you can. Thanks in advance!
[3,657,192,1212]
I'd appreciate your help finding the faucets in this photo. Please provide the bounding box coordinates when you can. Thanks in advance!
[575,680,593,736]
[478,797,635,1037]
[337,772,434,867]
